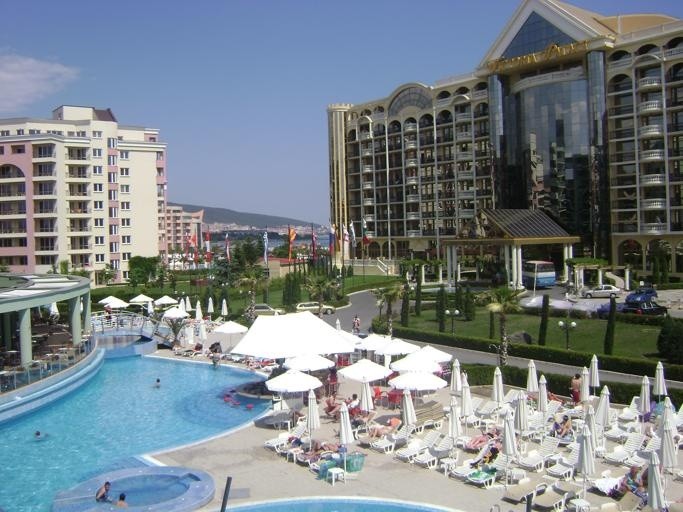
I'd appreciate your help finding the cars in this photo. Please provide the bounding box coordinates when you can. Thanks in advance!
[293,302,336,315]
[582,285,621,299]
[254,303,286,315]
[622,288,667,318]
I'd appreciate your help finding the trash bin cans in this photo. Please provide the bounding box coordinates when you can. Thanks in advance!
[346,454,365,472]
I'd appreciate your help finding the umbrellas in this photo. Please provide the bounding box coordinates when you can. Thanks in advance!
[50,293,248,355]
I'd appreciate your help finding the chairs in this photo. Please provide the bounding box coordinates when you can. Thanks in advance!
[264,388,683,512]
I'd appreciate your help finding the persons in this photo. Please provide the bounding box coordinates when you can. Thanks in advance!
[117,493,128,507]
[95,482,110,501]
[212,348,220,368]
[157,378,160,387]
[352,316,360,334]
[35,431,40,437]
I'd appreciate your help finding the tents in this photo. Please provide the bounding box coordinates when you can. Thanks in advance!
[231,310,354,357]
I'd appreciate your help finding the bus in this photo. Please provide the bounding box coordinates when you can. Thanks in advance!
[522,260,556,288]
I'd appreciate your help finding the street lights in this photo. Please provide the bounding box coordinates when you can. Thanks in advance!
[444,309,460,336]
[557,319,577,349]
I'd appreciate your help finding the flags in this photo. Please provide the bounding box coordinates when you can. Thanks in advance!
[263,233,268,266]
[192,234,198,264]
[185,238,190,256]
[225,234,231,261]
[205,233,212,262]
[312,232,318,262]
[330,218,372,257]
[288,230,297,262]
